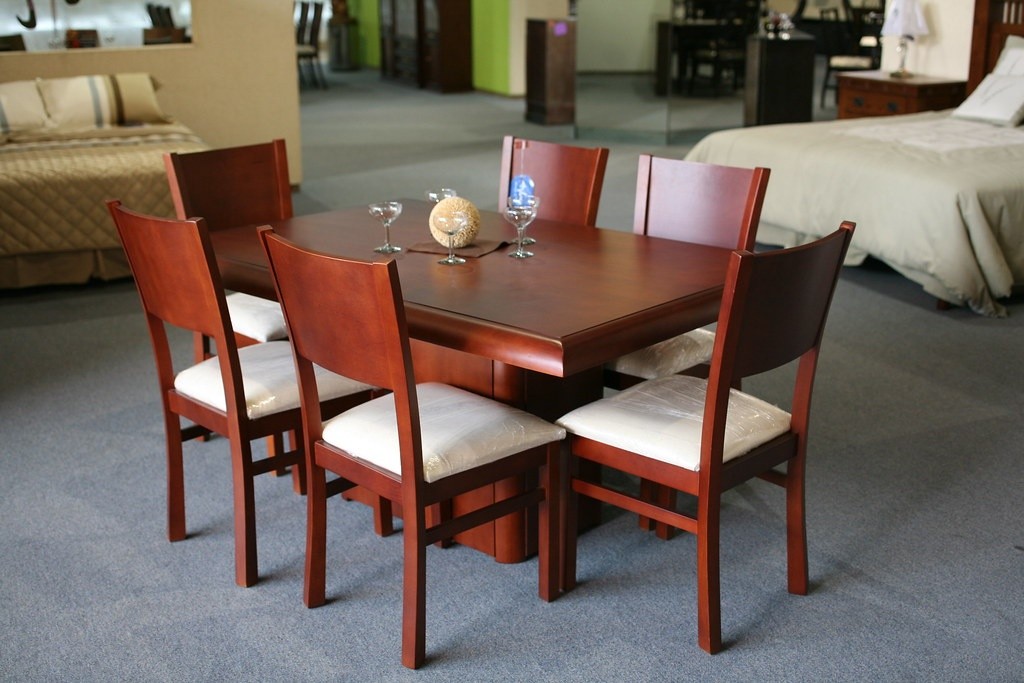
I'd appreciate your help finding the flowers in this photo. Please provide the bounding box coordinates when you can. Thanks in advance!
[762,13,794,30]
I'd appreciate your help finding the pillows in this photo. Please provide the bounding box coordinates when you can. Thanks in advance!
[951,75,1022,128]
[992,35,1023,75]
[0,71,172,131]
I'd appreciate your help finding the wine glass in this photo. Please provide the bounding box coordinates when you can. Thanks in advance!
[368,201,403,252]
[508,194,539,244]
[433,210,467,264]
[503,206,537,259]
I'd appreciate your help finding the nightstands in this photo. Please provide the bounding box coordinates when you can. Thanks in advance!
[836,72,968,121]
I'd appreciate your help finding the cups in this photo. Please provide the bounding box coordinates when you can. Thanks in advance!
[425,187,456,206]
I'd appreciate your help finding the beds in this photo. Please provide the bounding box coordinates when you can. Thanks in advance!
[683,0,1024,319]
[0,120,217,291]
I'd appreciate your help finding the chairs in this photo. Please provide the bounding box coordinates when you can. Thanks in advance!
[821,35,880,106]
[107,200,392,585]
[498,137,609,226]
[258,223,568,667]
[296,2,334,88]
[684,12,744,97]
[564,155,769,531]
[554,221,860,658]
[165,136,295,474]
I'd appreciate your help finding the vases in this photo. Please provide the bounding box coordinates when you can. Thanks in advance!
[770,27,785,36]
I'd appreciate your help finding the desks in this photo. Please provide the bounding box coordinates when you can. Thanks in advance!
[205,198,753,565]
[745,31,814,125]
[656,18,758,95]
